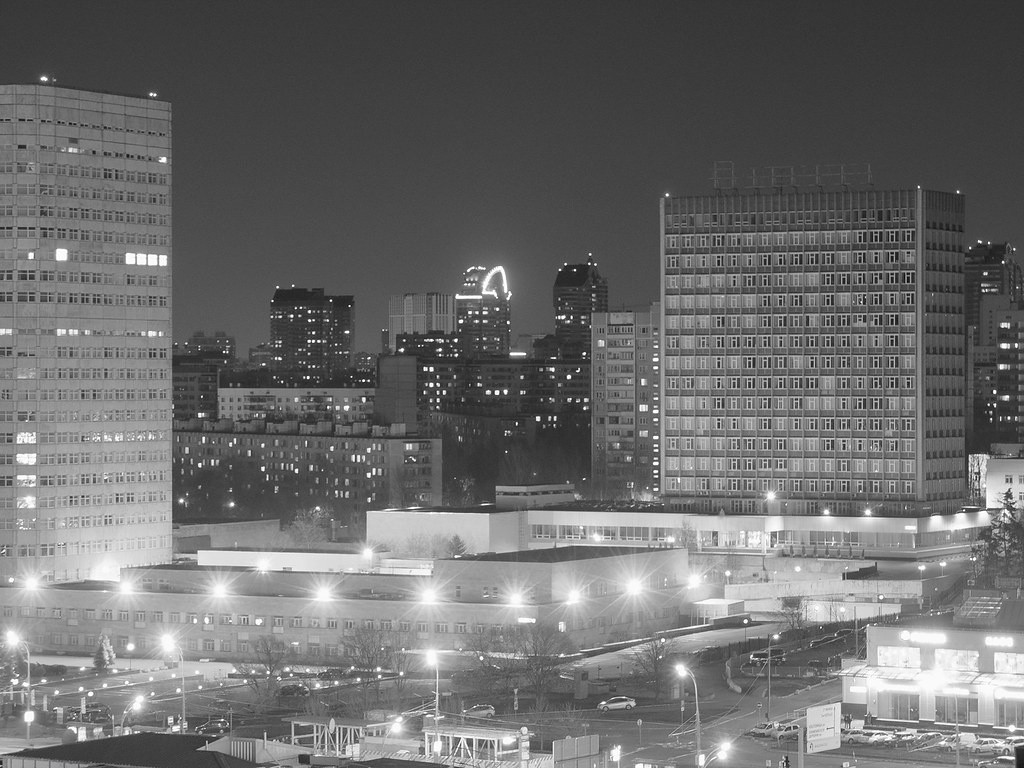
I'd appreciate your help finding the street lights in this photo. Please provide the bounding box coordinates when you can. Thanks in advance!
[424,649,442,718]
[699,741,731,767]
[767,633,780,721]
[5,631,33,742]
[162,633,186,735]
[124,642,134,670]
[674,661,705,768]
[120,694,145,737]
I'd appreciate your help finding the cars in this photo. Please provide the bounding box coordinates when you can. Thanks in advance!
[447,663,504,686]
[195,718,230,735]
[462,704,495,720]
[597,696,636,712]
[316,668,350,680]
[392,714,425,731]
[275,685,312,700]
[754,722,1024,757]
[69,702,113,724]
[0,700,27,724]
[751,626,867,668]
